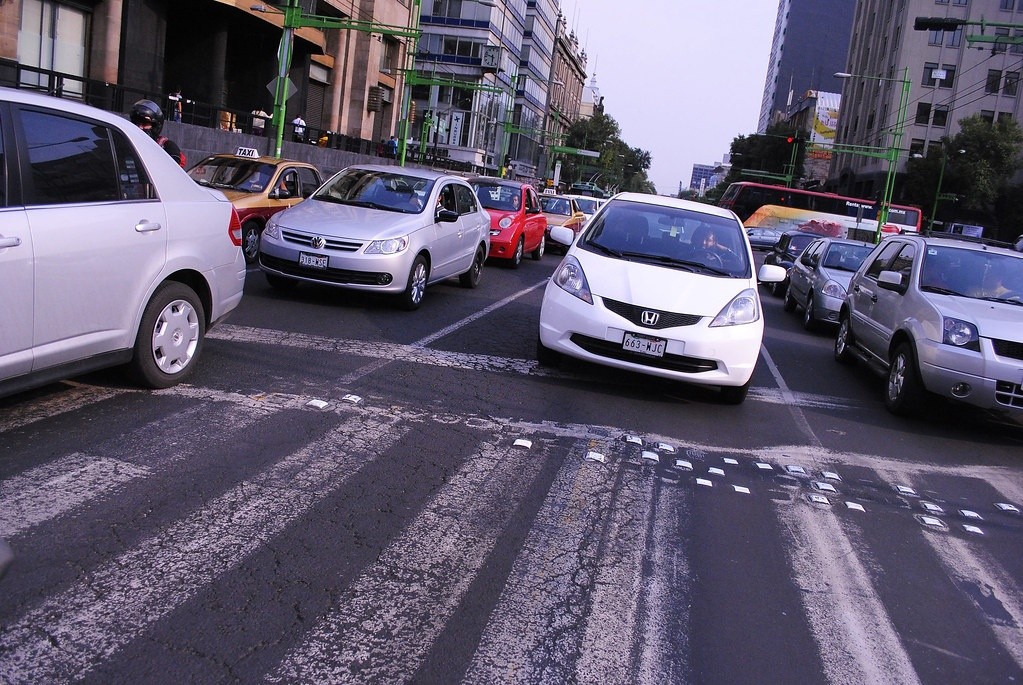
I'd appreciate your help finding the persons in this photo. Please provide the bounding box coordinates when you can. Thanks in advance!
[251,106,273,135]
[507,193,522,210]
[586,203,596,213]
[377,139,386,156]
[167,89,186,120]
[292,114,307,142]
[827,251,841,265]
[411,183,450,218]
[129,99,186,171]
[387,135,397,157]
[926,254,952,291]
[967,255,1021,299]
[564,204,572,215]
[681,225,744,272]
[791,240,799,252]
[238,172,291,199]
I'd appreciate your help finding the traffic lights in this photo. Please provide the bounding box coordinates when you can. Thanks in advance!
[546,179,553,187]
[505,154,512,167]
[787,137,804,143]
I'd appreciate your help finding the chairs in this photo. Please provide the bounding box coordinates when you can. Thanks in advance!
[827,250,841,266]
[478,190,491,202]
[614,213,650,248]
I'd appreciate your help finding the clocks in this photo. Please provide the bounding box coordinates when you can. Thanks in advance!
[484,47,500,66]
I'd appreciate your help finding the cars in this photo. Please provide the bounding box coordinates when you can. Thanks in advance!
[0,88,247,400]
[257,156,493,309]
[762,229,830,293]
[466,173,547,268]
[785,237,878,333]
[537,189,585,246]
[538,192,789,405]
[564,193,606,228]
[182,146,324,261]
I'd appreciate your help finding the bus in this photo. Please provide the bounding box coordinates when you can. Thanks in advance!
[712,182,922,252]
[571,182,602,198]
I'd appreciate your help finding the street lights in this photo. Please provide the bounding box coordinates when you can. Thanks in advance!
[433,99,472,163]
[497,76,565,177]
[912,149,966,231]
[833,73,913,246]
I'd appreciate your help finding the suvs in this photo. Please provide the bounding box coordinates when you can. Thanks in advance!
[834,224,1023,428]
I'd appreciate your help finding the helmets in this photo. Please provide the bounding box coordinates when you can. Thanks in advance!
[129,99,164,140]
